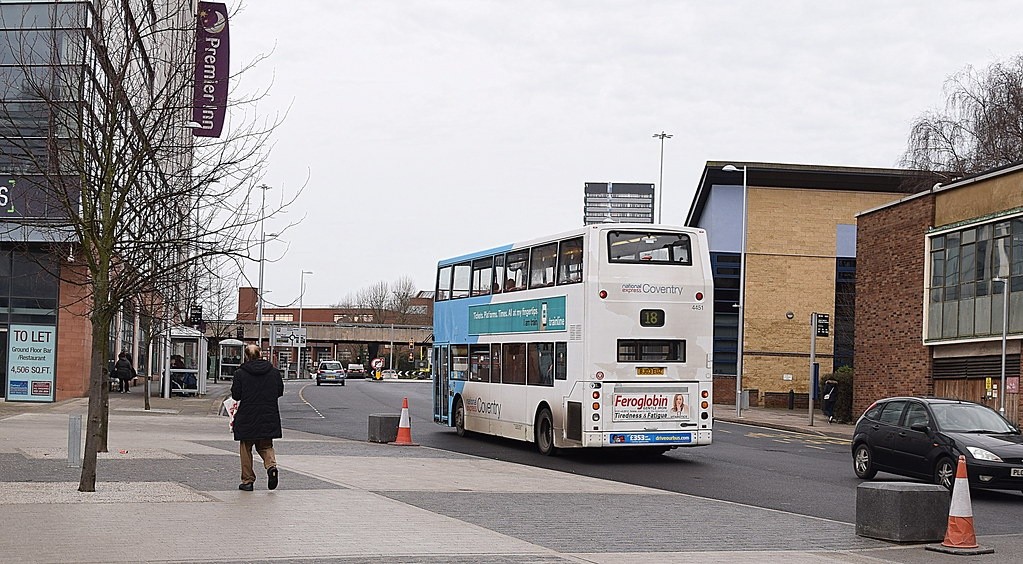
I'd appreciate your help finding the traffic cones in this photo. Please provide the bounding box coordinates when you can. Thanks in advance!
[924,455,996,556]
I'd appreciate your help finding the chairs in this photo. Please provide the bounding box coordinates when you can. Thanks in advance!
[110,371,119,391]
[453,277,582,300]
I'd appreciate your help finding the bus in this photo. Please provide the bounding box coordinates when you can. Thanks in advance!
[430,223,715,457]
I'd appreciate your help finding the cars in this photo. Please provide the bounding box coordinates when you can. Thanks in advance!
[316,360,346,387]
[381,369,399,379]
[850,395,1023,492]
[346,364,366,379]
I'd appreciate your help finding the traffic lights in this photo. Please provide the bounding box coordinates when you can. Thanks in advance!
[409,340,415,350]
[408,352,414,362]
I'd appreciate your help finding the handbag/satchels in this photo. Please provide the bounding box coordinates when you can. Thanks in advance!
[110,367,118,378]
[223,397,240,433]
[824,387,834,399]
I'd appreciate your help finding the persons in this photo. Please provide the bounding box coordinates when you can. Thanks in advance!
[507,279,515,292]
[163,356,199,397]
[486,282,499,294]
[822,377,839,423]
[110,346,134,394]
[207,351,211,379]
[671,394,689,416]
[217,354,241,381]
[230,343,285,491]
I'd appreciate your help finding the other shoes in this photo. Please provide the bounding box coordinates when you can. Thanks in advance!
[829,416,833,421]
[127,391,131,393]
[121,391,123,393]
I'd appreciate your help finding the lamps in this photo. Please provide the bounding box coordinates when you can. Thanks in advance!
[67,244,74,262]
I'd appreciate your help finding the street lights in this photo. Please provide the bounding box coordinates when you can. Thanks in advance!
[992,275,1009,419]
[296,269,313,379]
[256,183,274,348]
[162,121,204,397]
[721,162,748,420]
[387,396,421,446]
[652,130,673,224]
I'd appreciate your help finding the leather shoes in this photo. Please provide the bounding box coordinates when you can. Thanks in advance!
[267,467,278,490]
[239,482,253,491]
